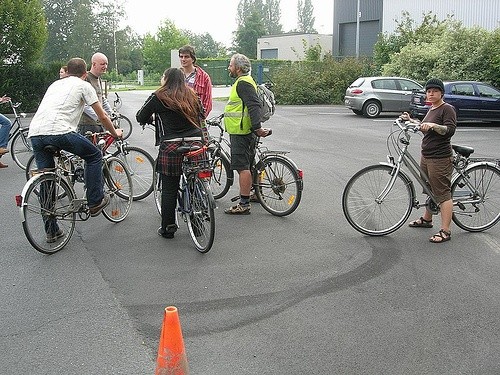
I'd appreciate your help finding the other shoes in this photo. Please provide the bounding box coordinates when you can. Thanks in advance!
[0,161,8,168]
[0,146,9,154]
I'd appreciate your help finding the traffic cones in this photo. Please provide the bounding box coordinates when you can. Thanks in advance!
[155,306,190,375]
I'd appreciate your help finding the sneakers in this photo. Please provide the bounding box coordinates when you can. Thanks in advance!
[88,193,110,218]
[193,223,207,237]
[225,205,253,215]
[45,227,65,243]
[250,193,266,202]
[157,227,174,239]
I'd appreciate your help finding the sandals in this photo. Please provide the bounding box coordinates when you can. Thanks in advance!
[409,217,433,228]
[430,229,451,243]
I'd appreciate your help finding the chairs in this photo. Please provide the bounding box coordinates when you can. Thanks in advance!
[383,81,391,88]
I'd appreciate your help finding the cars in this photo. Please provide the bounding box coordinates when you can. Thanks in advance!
[344,77,424,119]
[409,81,500,121]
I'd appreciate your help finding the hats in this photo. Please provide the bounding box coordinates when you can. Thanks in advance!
[424,78,445,94]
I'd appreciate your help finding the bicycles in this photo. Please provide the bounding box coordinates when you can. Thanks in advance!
[206,113,303,216]
[112,92,132,140]
[0,99,38,172]
[140,118,216,254]
[15,130,134,255]
[25,113,155,201]
[342,117,500,237]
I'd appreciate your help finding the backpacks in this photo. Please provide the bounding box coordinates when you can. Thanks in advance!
[240,75,276,123]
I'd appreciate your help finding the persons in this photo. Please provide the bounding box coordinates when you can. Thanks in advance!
[399,77,457,243]
[222,53,270,215]
[1,94,13,169]
[136,67,210,239]
[177,45,213,121]
[59,65,70,79]
[76,52,120,151]
[26,58,124,244]
